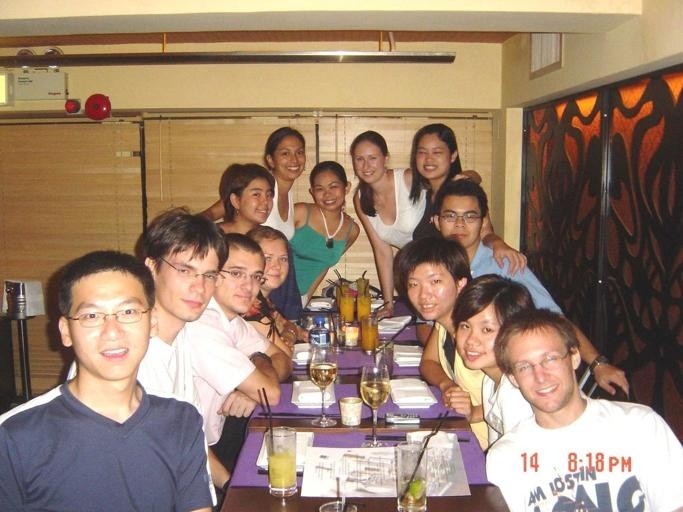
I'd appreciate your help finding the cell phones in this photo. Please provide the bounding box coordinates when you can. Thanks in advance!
[385,413,420,424]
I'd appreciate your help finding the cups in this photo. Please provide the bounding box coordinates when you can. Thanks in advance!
[396,441,427,512]
[333,277,379,355]
[318,500,358,511]
[372,338,395,377]
[338,395,363,428]
[265,426,298,498]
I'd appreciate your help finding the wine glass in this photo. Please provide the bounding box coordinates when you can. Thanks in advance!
[359,362,390,448]
[310,346,338,427]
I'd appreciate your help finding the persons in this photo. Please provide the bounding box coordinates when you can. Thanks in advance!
[393,177,629,452]
[486,308,683,510]
[67,206,335,495]
[1,250,218,511]
[195,123,527,326]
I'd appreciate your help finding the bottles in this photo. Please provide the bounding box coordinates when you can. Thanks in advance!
[310,318,331,365]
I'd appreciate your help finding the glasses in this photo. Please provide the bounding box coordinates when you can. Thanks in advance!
[439,212,482,223]
[507,350,572,378]
[160,257,225,286]
[220,269,268,286]
[67,308,150,327]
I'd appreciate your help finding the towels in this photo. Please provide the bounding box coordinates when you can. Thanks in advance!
[255,297,438,473]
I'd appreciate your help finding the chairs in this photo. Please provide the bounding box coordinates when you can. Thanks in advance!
[574,360,628,402]
[0,279,32,416]
